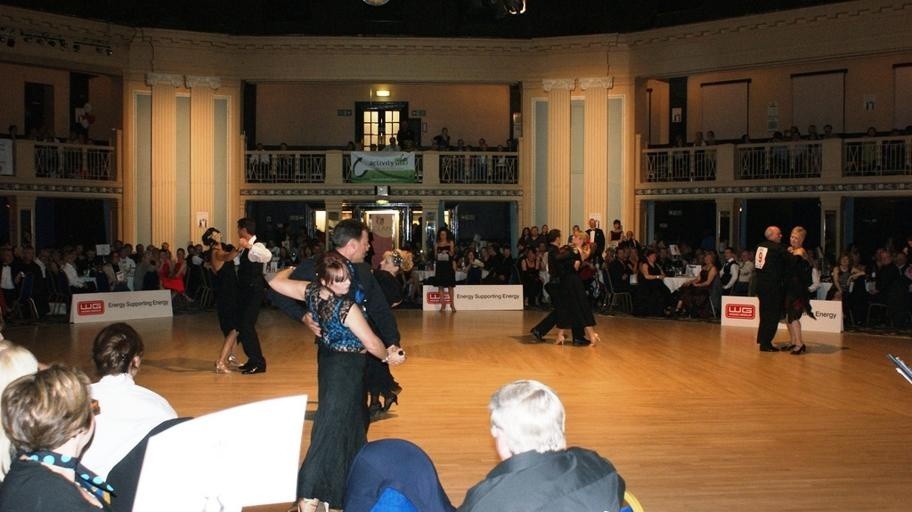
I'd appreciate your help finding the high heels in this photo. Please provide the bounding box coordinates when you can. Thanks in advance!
[553,335,565,344]
[368,392,398,412]
[588,332,600,347]
[286,497,344,512]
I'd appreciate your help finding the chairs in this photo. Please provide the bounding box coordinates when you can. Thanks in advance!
[44,273,69,318]
[600,267,634,313]
[198,267,213,307]
[12,272,39,321]
[177,266,193,303]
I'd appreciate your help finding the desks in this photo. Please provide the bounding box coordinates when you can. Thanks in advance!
[628,272,700,294]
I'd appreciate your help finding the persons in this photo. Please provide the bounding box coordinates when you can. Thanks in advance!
[342,437,456,512]
[29,112,64,178]
[270,142,294,182]
[530,229,600,347]
[754,225,819,355]
[250,143,270,182]
[570,219,755,322]
[826,239,910,333]
[64,114,95,179]
[268,236,322,270]
[1,360,115,512]
[268,220,414,512]
[1,240,218,327]
[456,379,625,512]
[0,340,39,479]
[435,226,549,313]
[343,120,518,183]
[667,124,911,180]
[199,220,272,376]
[79,322,178,483]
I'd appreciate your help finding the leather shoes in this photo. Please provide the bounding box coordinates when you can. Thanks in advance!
[760,344,805,354]
[214,354,266,374]
[531,328,546,342]
[573,336,591,346]
